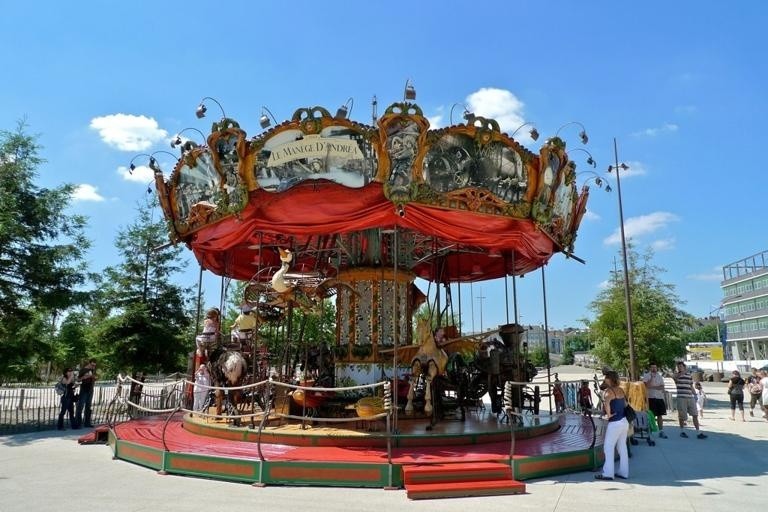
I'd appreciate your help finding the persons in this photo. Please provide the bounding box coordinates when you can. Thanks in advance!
[553,380,567,413]
[576,361,709,439]
[75,358,96,428]
[727,370,745,422]
[433,326,451,344]
[57,367,80,430]
[196,310,219,357]
[594,371,630,480]
[229,306,257,343]
[759,369,768,422]
[747,367,765,417]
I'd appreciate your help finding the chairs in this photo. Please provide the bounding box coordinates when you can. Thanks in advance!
[680,432,688,439]
[614,474,629,480]
[659,431,668,439]
[594,474,614,480]
[697,433,708,439]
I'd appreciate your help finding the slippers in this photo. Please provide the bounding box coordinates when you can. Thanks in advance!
[730,382,743,395]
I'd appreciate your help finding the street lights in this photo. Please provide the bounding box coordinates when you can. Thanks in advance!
[619,387,636,424]
[55,382,65,395]
